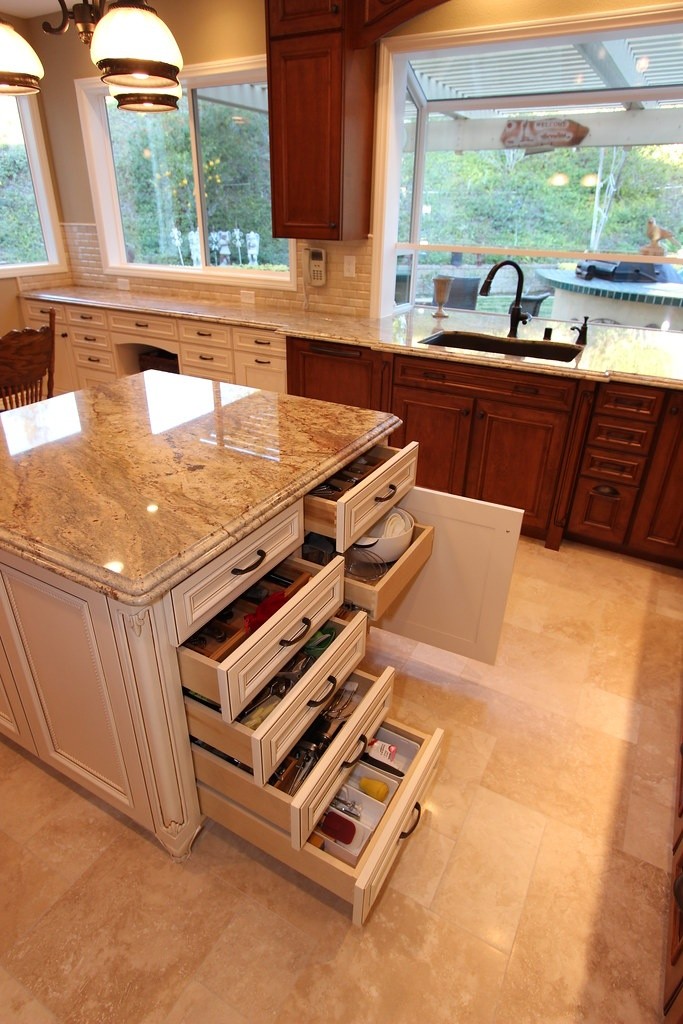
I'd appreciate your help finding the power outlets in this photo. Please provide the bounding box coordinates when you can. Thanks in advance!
[117,279,130,291]
[240,290,255,304]
[343,256,357,279]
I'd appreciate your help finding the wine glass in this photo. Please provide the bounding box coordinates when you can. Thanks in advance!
[433,278,455,317]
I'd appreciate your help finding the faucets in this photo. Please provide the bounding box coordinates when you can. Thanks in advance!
[477,259,533,338]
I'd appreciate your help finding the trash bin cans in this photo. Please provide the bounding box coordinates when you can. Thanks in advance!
[433,272,481,311]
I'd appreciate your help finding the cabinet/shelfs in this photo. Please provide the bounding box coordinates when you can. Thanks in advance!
[18,295,285,410]
[1,442,525,928]
[566,381,683,565]
[388,353,597,552]
[286,337,394,413]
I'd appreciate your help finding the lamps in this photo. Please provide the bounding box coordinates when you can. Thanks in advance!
[0,0,183,113]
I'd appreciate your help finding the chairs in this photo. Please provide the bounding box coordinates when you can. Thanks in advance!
[0,310,61,411]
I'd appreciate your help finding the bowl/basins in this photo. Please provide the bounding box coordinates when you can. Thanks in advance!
[349,508,416,563]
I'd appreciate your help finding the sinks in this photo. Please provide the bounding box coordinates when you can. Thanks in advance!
[417,329,585,363]
